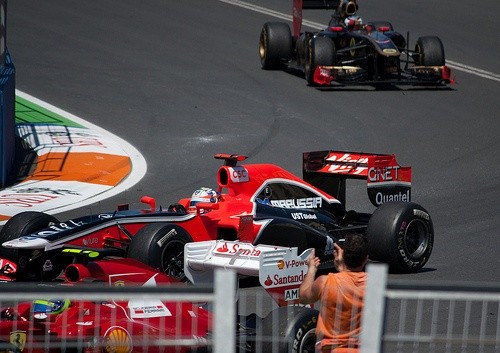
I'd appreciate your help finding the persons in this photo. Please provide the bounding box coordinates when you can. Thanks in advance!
[188,187,218,214]
[336,0,363,26]
[300,234,368,353]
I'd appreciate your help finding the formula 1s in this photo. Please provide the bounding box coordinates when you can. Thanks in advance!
[0,150,434,353]
[259,0,454,91]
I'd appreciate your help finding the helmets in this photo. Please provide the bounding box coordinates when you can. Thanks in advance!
[187,187,218,214]
[344,14,362,27]
[30,298,71,320]
